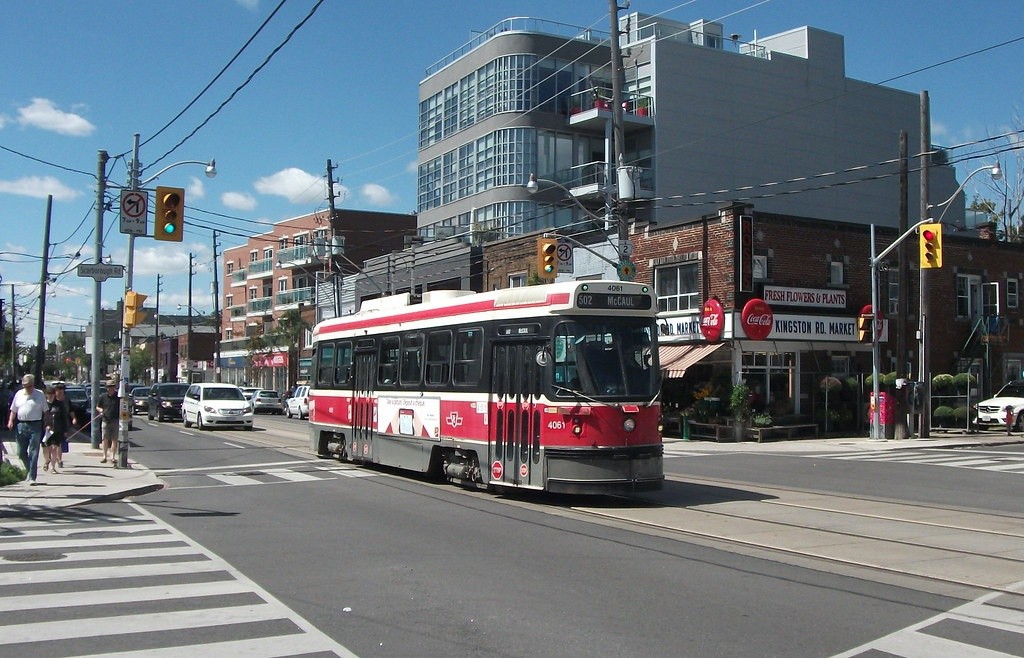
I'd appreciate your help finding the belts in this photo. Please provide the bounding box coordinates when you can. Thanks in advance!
[20,420,42,423]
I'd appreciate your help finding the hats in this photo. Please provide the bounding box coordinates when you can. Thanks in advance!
[106,380,116,387]
[1006,405,1013,409]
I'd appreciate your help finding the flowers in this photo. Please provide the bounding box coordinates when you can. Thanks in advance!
[752,411,773,427]
[693,379,713,399]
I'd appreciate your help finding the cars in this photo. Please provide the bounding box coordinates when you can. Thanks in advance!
[284,385,310,420]
[43,381,151,428]
[181,383,254,431]
[239,386,283,416]
[149,383,190,422]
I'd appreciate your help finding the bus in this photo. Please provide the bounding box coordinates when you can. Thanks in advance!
[308,279,665,497]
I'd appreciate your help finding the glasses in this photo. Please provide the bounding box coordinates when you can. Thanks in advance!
[109,385,115,388]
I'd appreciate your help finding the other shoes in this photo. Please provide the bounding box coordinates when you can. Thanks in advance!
[111,459,117,463]
[26,472,30,481]
[51,468,57,474]
[100,459,107,464]
[58,461,64,468]
[29,480,36,486]
[43,460,50,472]
[1008,434,1013,436]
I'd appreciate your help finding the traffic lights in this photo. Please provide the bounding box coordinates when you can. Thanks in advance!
[154,186,185,242]
[125,291,148,327]
[919,223,943,269]
[858,317,873,342]
[537,238,557,278]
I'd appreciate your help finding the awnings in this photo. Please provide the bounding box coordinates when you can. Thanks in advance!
[634,343,724,378]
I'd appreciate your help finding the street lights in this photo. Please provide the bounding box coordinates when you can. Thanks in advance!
[918,160,1002,438]
[153,314,192,383]
[527,173,630,282]
[117,134,217,467]
[177,304,221,383]
[10,284,56,390]
[34,194,112,388]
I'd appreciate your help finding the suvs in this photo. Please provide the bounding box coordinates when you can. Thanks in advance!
[972,381,1024,432]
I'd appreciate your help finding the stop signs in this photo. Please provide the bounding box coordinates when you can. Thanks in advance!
[491,461,503,481]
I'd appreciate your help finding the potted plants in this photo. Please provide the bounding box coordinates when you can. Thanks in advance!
[931,373,976,428]
[730,379,751,423]
[592,89,606,109]
[571,100,582,114]
[692,397,724,423]
[864,371,897,396]
[635,97,649,116]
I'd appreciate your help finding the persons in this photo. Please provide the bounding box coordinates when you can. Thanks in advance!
[7,374,52,485]
[40,384,77,474]
[95,381,119,465]
